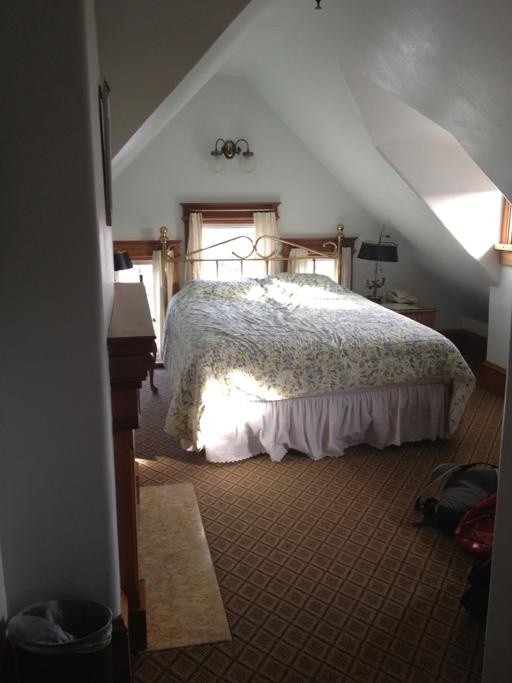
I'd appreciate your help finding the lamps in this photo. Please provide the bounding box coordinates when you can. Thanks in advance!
[114,248,134,271]
[355,229,399,304]
[208,136,256,173]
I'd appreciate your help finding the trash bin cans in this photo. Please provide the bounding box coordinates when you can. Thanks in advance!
[7,598,114,683]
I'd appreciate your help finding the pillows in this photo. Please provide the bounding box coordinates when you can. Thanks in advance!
[182,272,349,302]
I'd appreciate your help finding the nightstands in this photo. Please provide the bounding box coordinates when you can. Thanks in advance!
[377,298,440,330]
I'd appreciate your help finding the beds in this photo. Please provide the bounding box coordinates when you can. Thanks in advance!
[159,224,451,464]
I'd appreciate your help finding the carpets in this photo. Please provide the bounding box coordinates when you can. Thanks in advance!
[135,480,233,651]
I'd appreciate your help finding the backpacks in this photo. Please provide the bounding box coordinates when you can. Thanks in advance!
[411,461,498,526]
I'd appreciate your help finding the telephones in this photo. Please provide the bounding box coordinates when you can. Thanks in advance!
[386,288,418,305]
